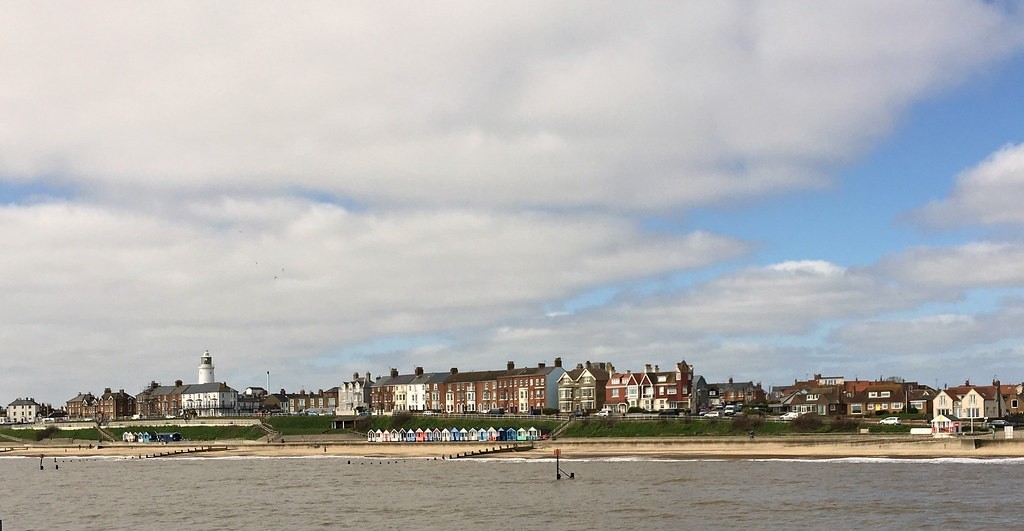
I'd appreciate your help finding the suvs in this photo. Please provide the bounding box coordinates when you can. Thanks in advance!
[594,410,612,417]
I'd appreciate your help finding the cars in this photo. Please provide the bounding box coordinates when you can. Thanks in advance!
[704,411,720,418]
[988,420,1012,427]
[716,404,723,409]
[2,420,12,424]
[569,412,586,417]
[165,413,175,418]
[698,409,707,415]
[736,404,743,412]
[423,410,438,417]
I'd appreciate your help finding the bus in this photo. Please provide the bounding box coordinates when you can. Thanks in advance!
[659,408,691,418]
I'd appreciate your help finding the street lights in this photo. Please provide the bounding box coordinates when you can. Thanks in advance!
[267,371,269,394]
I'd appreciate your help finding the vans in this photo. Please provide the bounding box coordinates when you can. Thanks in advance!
[779,413,802,420]
[359,413,371,416]
[880,417,901,425]
[488,408,504,416]
[131,415,143,420]
[724,405,736,415]
